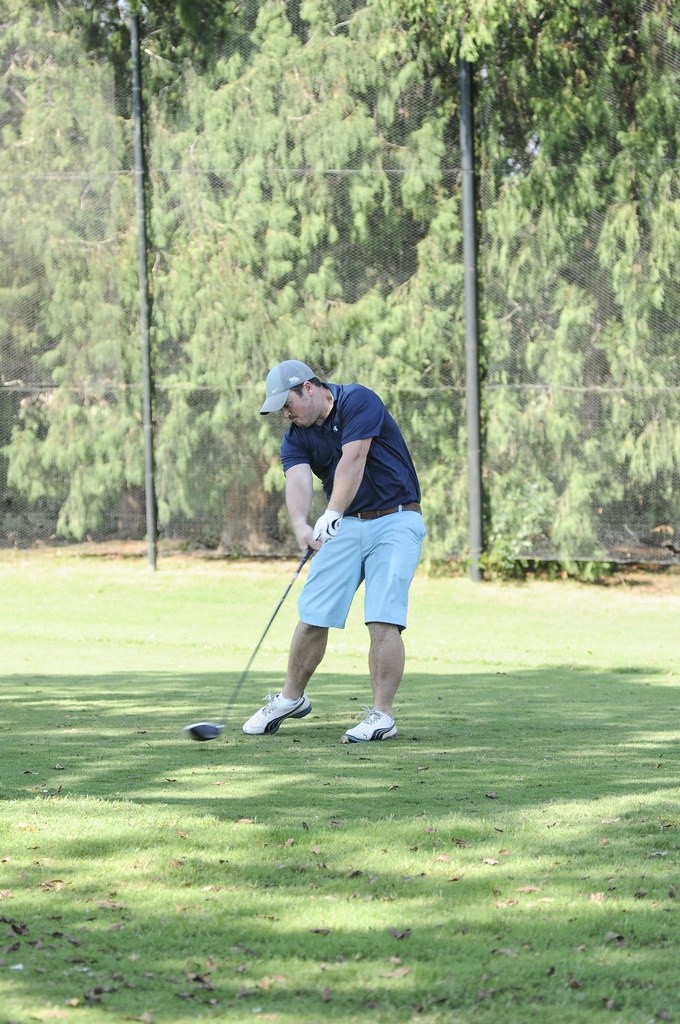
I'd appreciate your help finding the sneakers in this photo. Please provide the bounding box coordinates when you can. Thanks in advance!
[243,689,312,736]
[344,705,397,743]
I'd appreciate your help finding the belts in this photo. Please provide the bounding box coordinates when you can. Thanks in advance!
[344,503,421,520]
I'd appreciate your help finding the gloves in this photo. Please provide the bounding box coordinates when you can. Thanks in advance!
[312,510,343,543]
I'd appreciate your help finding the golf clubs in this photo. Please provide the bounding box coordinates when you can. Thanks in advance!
[183,547,313,741]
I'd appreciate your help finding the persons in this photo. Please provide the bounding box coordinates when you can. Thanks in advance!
[241,359,427,742]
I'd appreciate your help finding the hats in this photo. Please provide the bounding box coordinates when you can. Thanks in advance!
[260,360,315,415]
